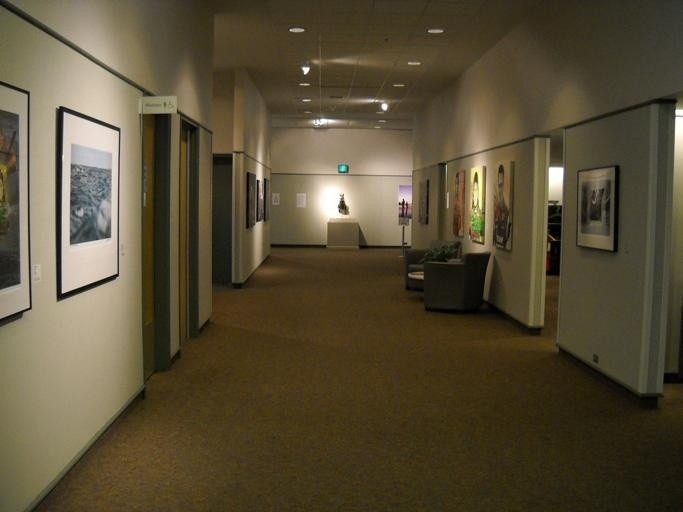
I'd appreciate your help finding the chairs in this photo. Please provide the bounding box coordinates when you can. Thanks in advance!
[405,241,492,311]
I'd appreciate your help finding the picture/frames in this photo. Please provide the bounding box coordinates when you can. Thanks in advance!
[576,165,619,252]
[0,77,122,321]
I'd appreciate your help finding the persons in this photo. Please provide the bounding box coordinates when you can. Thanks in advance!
[451,171,462,236]
[491,165,509,249]
[467,171,482,243]
[405,202,408,209]
[401,198,405,209]
